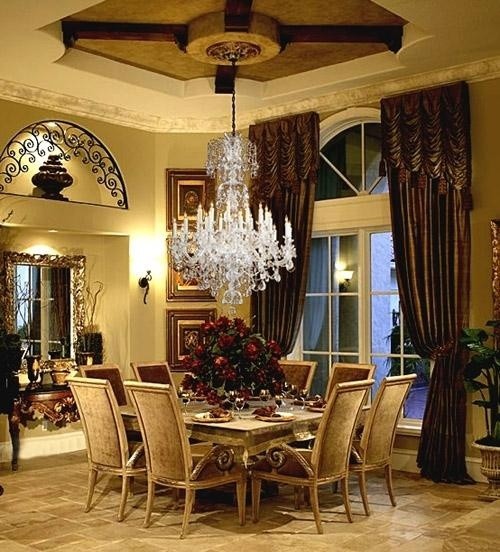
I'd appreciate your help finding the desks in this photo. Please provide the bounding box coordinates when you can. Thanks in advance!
[1,385,80,470]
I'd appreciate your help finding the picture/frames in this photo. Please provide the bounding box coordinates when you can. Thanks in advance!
[164,167,218,372]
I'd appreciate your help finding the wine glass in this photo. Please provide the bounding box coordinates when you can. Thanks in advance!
[232,381,310,420]
[181,393,191,417]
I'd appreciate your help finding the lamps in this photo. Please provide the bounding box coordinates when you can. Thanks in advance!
[165,53,298,305]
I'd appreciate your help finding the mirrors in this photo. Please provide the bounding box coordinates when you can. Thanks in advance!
[3,250,87,373]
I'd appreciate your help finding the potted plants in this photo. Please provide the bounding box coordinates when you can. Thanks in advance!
[458,320,500,501]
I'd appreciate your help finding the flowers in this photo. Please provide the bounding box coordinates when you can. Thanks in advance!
[177,315,289,404]
